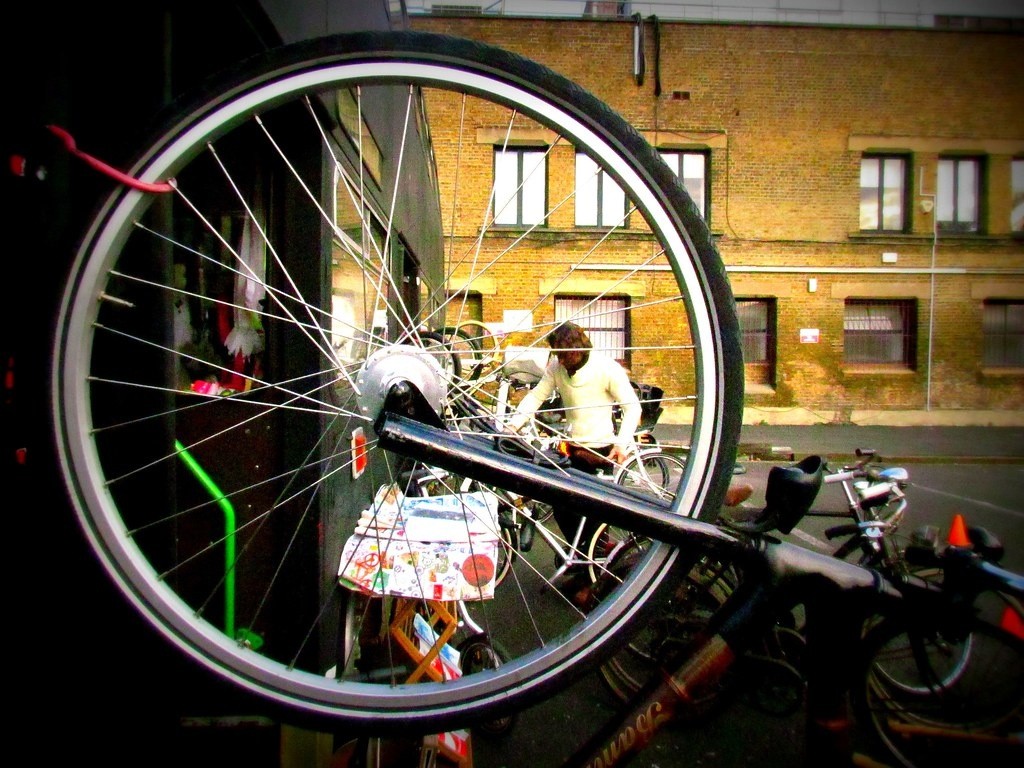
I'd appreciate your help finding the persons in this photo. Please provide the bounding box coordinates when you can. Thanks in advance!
[499,322,643,600]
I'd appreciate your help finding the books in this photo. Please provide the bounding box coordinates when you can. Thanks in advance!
[413,613,463,681]
[437,729,470,762]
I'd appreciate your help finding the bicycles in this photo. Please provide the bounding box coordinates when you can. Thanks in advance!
[45,29,1024,767]
